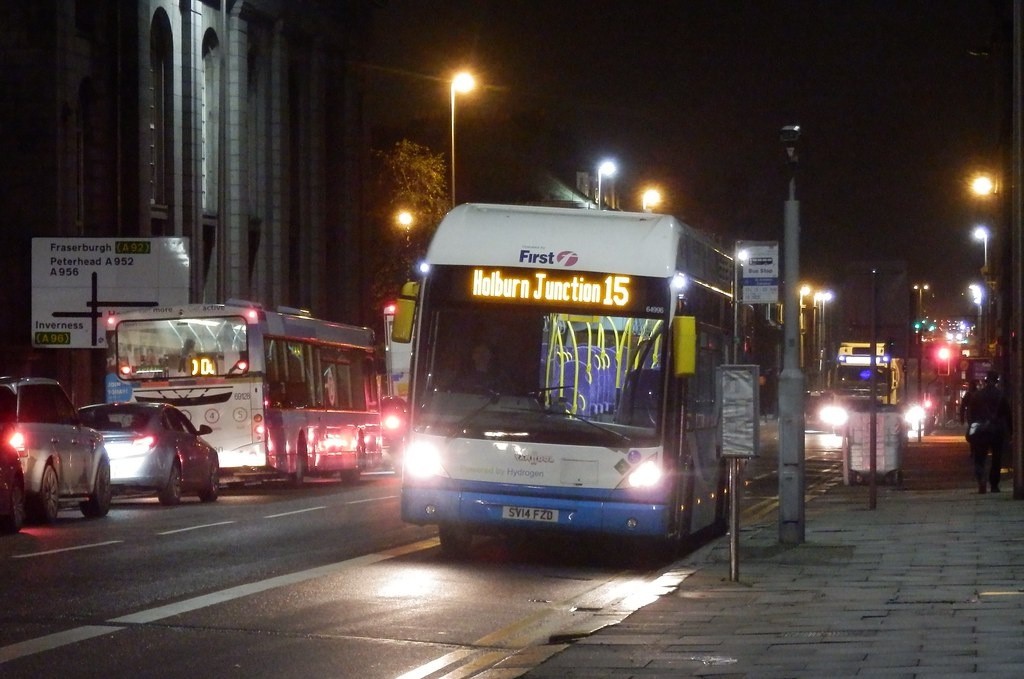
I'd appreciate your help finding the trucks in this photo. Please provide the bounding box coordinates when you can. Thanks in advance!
[834,341,903,406]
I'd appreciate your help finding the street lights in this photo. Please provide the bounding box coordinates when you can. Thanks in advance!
[597,163,613,210]
[449,73,474,209]
[914,282,931,441]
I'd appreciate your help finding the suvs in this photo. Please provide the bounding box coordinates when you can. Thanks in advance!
[0,375,115,534]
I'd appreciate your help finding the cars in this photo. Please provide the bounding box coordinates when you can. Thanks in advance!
[77,402,220,505]
[0,437,26,531]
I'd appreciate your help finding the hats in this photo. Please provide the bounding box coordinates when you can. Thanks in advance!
[984,371,1000,383]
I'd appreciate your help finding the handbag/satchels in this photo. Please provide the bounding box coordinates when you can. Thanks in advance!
[966,420,985,445]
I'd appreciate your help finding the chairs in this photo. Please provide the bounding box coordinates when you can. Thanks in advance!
[538,342,662,418]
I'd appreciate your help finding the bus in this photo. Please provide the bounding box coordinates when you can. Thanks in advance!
[107,299,386,489]
[391,202,756,551]
[381,302,420,409]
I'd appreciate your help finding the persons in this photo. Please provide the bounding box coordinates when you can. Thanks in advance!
[452,342,515,396]
[958,370,1015,494]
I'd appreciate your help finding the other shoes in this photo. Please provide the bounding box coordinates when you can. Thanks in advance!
[976,482,987,493]
[991,485,1000,493]
